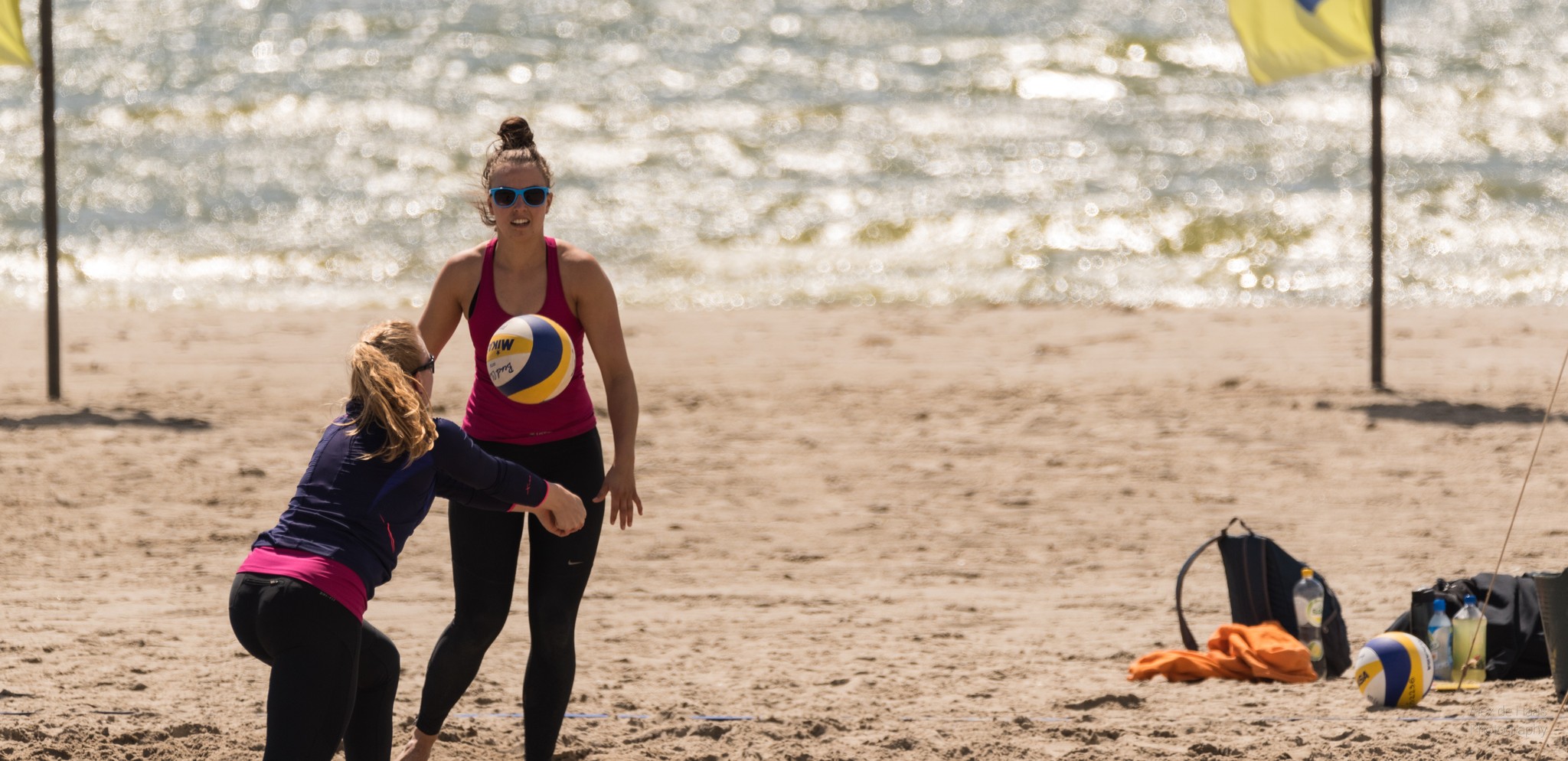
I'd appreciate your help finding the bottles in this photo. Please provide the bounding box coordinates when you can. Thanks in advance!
[1452,595,1487,683]
[1293,568,1328,683]
[1427,599,1454,681]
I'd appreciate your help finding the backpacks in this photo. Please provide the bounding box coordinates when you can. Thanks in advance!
[1174,516,1353,678]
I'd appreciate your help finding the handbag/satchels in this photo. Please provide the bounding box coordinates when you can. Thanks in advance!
[1411,569,1551,680]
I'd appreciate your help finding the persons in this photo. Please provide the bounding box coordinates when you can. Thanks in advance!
[394,118,644,761]
[227,321,587,761]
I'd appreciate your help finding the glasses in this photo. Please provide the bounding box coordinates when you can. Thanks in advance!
[488,187,550,206]
[413,355,436,390]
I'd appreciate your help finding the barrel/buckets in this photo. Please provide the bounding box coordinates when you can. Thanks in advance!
[1533,567,1568,705]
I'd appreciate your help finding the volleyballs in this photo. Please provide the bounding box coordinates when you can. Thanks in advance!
[486,313,577,406]
[1355,632,1433,707]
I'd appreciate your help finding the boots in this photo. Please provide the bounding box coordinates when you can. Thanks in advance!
[1532,571,1568,705]
